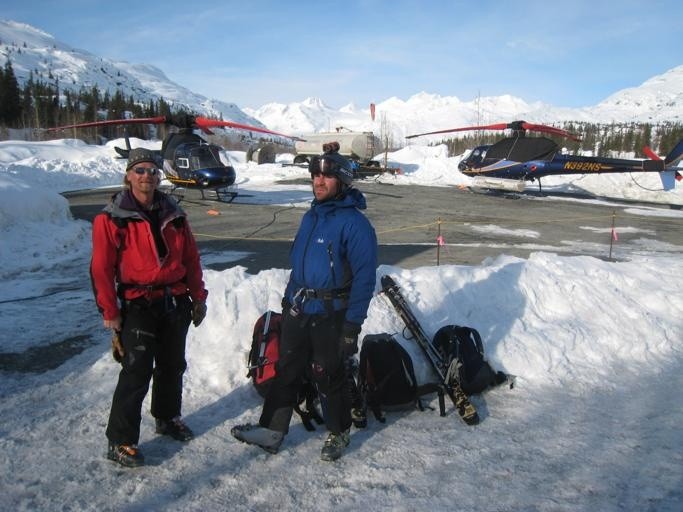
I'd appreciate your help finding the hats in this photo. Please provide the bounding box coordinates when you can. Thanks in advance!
[126,147,158,170]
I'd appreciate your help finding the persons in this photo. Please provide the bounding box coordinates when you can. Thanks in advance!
[89,148,209,465]
[231,152,379,461]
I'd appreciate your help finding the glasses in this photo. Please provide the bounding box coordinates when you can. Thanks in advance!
[309,156,341,175]
[134,166,157,175]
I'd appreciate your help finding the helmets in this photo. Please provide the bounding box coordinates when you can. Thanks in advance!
[308,151,353,185]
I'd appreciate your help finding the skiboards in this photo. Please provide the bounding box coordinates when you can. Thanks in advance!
[348,275,480,428]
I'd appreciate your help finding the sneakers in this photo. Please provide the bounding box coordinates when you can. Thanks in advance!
[155,416,194,442]
[108,442,145,467]
[231,423,284,455]
[321,430,350,462]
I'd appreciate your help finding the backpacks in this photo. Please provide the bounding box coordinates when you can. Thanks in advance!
[357,333,446,424]
[246,311,324,431]
[432,324,504,395]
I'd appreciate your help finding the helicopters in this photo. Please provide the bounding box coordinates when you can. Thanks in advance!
[404,120,683,198]
[43,115,311,206]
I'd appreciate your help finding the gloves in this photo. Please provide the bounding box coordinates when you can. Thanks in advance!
[191,302,207,327]
[113,332,126,363]
[336,321,362,367]
